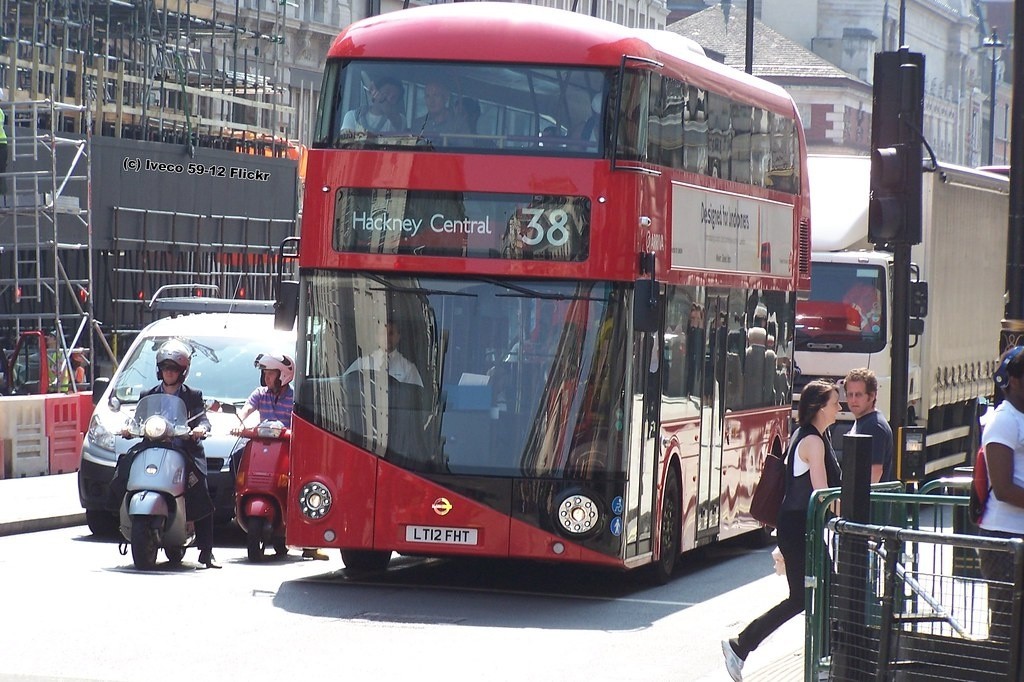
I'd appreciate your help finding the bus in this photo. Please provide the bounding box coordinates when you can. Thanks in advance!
[276,0,815,587]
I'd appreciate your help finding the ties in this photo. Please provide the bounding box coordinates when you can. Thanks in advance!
[381,355,390,374]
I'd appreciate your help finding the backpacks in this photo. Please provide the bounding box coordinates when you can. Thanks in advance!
[969,406,1019,524]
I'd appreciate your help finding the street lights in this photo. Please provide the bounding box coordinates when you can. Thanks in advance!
[983,26,1005,166]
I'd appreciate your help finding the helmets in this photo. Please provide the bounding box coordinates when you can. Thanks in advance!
[259,353,295,388]
[156,342,189,381]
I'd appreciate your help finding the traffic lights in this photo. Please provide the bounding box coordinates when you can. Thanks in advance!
[868,144,922,246]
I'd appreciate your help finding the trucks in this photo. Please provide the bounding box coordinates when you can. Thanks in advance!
[805,152,1009,510]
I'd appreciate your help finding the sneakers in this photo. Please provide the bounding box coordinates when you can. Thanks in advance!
[722,638,745,682]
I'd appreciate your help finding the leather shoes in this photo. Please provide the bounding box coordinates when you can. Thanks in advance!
[198,550,222,569]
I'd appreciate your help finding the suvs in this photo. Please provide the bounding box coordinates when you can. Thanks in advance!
[79,282,325,537]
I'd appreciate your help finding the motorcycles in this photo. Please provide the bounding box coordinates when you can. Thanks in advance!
[221,402,293,562]
[110,396,216,569]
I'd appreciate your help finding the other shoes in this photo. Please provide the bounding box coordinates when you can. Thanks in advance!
[302,548,329,561]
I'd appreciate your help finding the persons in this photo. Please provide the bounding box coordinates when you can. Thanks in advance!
[232,352,330,560]
[343,319,424,387]
[412,81,500,148]
[977,346,1024,646]
[633,302,793,413]
[0,87,8,196]
[340,77,407,138]
[19,330,69,393]
[68,348,87,392]
[721,381,843,682]
[842,272,880,334]
[542,126,564,148]
[568,90,639,154]
[843,368,893,604]
[486,340,535,407]
[105,340,222,568]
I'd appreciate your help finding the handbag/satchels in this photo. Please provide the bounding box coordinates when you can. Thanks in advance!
[751,446,789,528]
